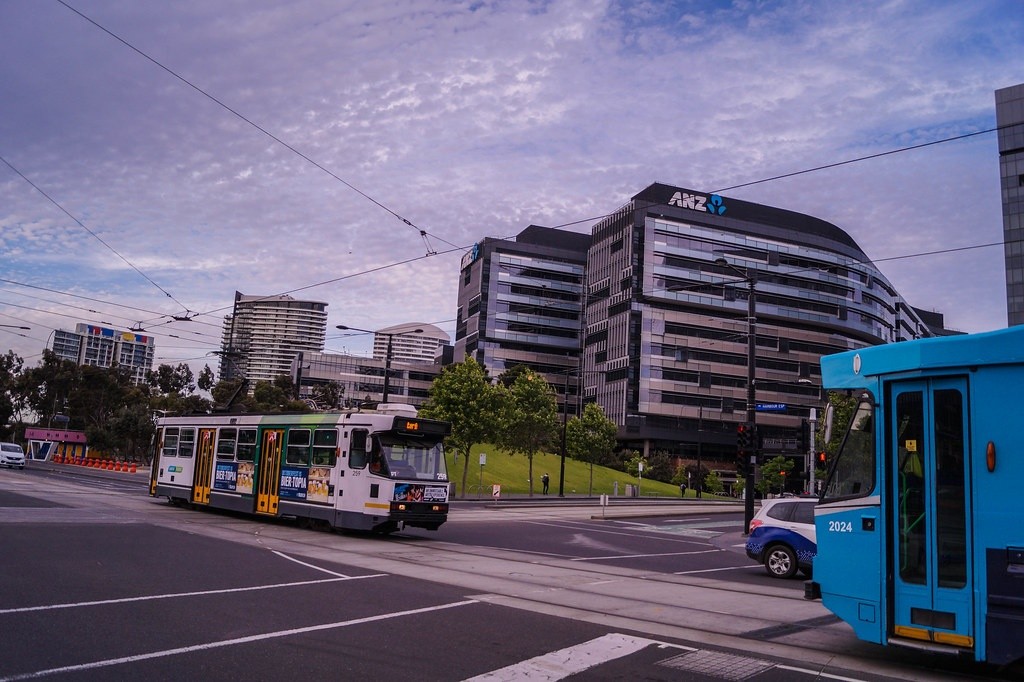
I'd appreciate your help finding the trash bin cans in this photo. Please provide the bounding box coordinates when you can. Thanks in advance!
[625,483,638,498]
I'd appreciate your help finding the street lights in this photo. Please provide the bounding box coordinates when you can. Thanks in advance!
[336,325,424,403]
[668,257,756,538]
[558,369,615,496]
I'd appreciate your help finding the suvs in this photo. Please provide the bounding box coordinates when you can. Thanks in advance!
[746,497,819,579]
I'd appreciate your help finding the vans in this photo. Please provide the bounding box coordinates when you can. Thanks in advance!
[1,442,25,469]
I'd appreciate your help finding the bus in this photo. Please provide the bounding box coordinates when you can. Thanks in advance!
[804,325,1024,664]
[149,402,460,534]
